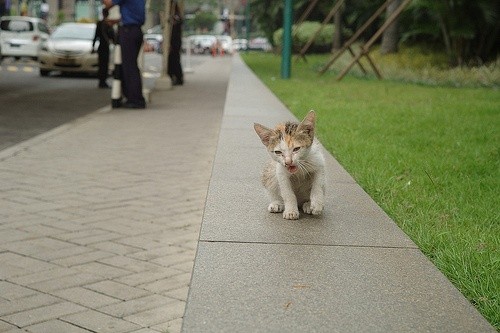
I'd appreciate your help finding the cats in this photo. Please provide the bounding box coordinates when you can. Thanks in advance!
[253,109,326,220]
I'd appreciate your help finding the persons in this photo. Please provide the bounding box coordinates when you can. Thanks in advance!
[102,0,146,109]
[166,0,184,86]
[89,8,114,87]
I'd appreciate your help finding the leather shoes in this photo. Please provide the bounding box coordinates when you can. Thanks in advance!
[119,98,146,108]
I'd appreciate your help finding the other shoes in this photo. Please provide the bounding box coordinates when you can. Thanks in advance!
[98,80,113,88]
[173,80,184,86]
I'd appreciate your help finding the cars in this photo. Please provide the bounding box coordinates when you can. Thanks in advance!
[139,27,275,58]
[37,21,120,80]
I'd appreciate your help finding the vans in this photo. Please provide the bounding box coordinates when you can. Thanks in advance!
[0,14,51,62]
